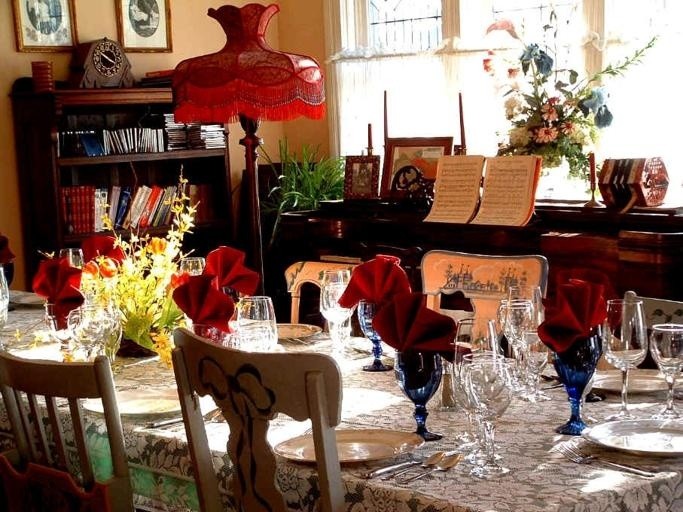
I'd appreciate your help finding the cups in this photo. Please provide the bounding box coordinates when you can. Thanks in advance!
[29,60,54,92]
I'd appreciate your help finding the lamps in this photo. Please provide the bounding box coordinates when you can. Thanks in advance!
[166,3,327,310]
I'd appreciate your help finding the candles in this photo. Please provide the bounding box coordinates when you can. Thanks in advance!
[383,90,388,142]
[367,121,373,148]
[458,92,466,145]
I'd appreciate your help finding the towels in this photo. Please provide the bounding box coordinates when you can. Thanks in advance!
[28,255,84,331]
[337,253,474,363]
[171,272,237,338]
[79,233,125,275]
[535,275,611,354]
[201,245,262,296]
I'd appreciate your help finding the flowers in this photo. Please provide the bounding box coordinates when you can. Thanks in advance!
[469,3,659,156]
[13,189,201,372]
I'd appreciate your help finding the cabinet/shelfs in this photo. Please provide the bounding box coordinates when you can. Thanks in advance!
[8,77,238,293]
[316,197,683,302]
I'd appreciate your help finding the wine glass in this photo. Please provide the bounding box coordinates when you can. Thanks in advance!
[599,298,648,419]
[59,247,84,269]
[549,331,594,437]
[648,322,683,418]
[391,348,445,442]
[223,290,284,354]
[451,282,551,475]
[67,300,123,362]
[0,266,11,350]
[178,255,207,278]
[319,267,393,373]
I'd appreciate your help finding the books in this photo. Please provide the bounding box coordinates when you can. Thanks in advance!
[55,109,228,239]
[468,156,543,227]
[420,154,486,228]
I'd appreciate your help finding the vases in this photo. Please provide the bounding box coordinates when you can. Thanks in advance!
[537,149,595,205]
[117,328,159,357]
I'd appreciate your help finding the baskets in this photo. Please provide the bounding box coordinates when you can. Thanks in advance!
[114,328,161,359]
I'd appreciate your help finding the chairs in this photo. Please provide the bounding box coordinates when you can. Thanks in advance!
[417,248,551,346]
[618,288,682,347]
[280,256,371,339]
[170,325,344,511]
[0,347,137,511]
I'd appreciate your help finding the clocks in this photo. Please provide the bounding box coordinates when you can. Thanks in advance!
[69,38,135,88]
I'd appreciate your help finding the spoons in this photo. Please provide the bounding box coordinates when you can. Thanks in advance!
[146,407,222,427]
[380,450,464,486]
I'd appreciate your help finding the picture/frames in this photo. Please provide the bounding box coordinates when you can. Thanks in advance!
[342,153,381,201]
[114,0,172,54]
[12,0,77,55]
[378,136,453,197]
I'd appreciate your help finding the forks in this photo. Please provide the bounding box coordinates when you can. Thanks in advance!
[553,440,655,480]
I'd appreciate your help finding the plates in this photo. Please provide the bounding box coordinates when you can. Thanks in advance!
[273,320,324,340]
[81,388,190,421]
[578,417,683,456]
[13,293,46,305]
[585,366,683,395]
[273,428,427,463]
